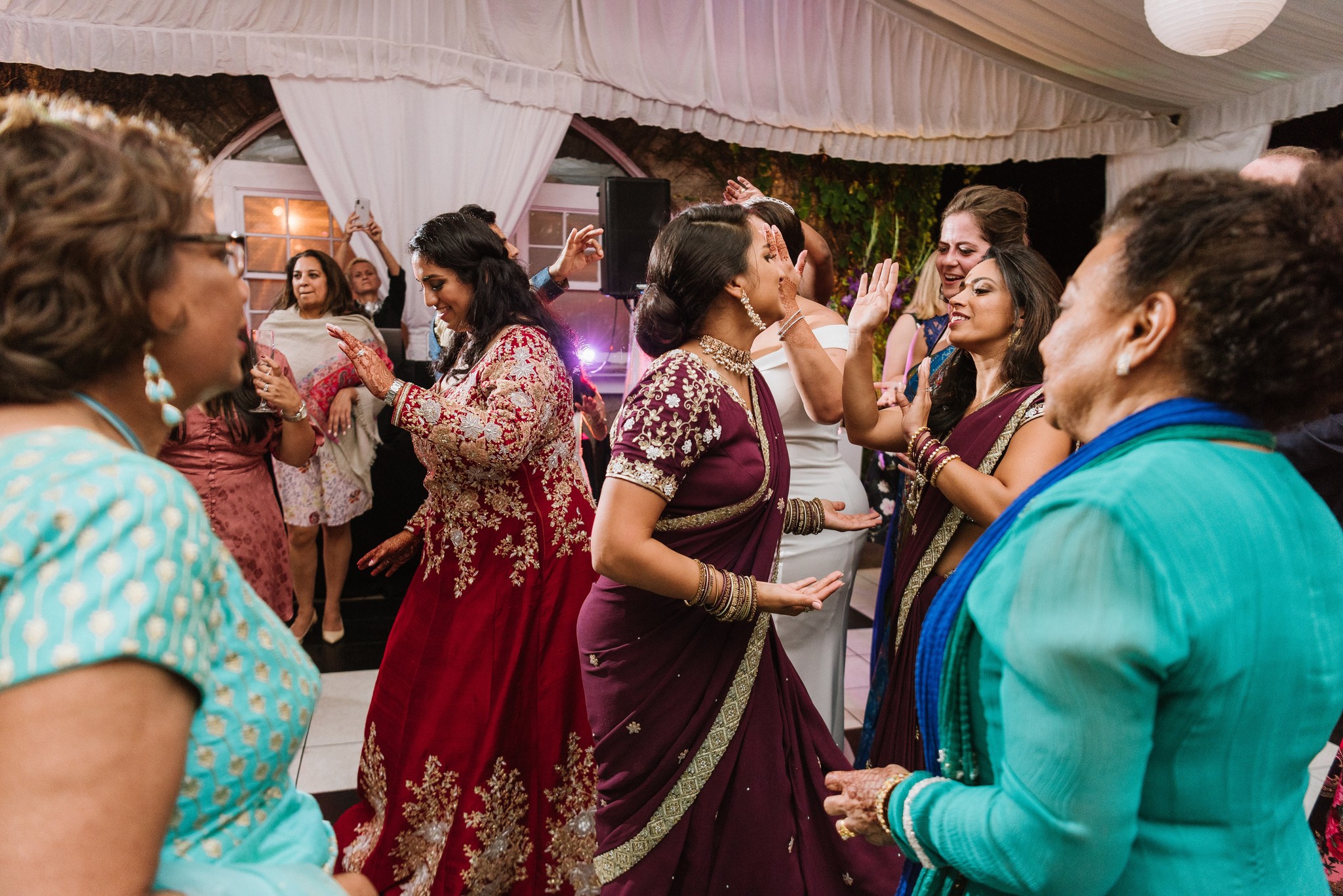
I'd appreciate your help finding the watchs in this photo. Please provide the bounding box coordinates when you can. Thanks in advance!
[280,399,308,421]
[384,378,406,406]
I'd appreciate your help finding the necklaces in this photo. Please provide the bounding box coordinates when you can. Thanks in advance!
[66,389,150,452]
[693,334,754,376]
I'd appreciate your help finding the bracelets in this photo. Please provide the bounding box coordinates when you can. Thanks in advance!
[873,773,910,836]
[783,497,824,535]
[778,308,801,335]
[683,558,758,623]
[780,315,804,342]
[908,426,962,487]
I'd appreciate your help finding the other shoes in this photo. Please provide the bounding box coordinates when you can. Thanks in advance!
[289,608,320,642]
[321,608,347,645]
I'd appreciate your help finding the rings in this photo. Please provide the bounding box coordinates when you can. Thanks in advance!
[356,349,365,357]
[925,387,933,392]
[263,384,269,392]
[804,607,815,613]
[838,822,857,842]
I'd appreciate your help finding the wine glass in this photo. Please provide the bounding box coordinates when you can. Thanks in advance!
[248,329,278,413]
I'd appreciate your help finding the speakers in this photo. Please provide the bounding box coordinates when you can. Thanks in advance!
[598,177,671,299]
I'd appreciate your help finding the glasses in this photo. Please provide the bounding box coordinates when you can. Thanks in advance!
[152,225,250,278]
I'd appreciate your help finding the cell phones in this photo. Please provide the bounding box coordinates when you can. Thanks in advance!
[353,198,370,229]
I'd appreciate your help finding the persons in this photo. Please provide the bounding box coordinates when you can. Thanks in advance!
[573,200,907,896]
[875,184,1029,411]
[571,357,610,504]
[0,85,382,895]
[722,195,871,749]
[326,212,602,896]
[334,206,406,372]
[427,204,603,383]
[253,250,390,644]
[725,176,834,305]
[159,334,316,621]
[825,151,1341,895]
[841,240,1084,771]
